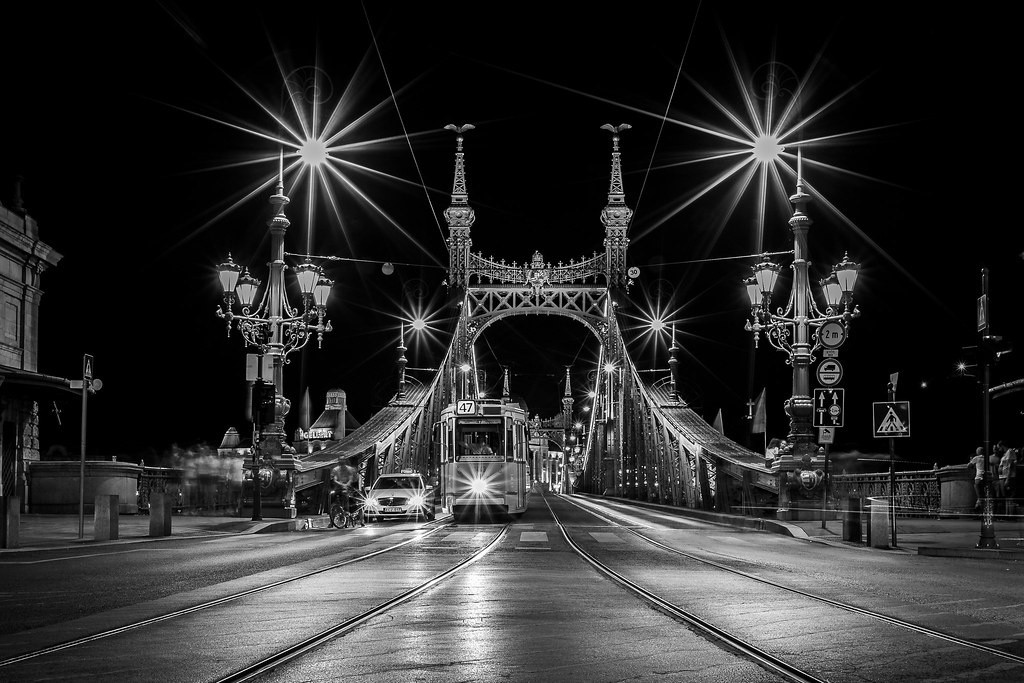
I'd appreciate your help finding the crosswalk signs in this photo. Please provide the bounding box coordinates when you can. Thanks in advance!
[872,373,911,547]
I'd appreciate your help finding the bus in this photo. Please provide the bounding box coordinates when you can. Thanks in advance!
[431,398,531,521]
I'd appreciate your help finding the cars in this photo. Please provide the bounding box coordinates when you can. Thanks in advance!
[361,468,436,523]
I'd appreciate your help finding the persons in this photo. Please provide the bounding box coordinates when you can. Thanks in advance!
[967,439,1017,511]
[327,455,365,529]
[455,435,494,455]
[170,444,246,516]
[765,438,790,459]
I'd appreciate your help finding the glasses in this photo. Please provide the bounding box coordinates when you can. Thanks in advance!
[339,459,346,460]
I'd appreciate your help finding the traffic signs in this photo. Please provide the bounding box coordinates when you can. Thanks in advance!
[813,321,846,529]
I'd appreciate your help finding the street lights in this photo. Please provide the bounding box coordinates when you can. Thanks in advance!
[603,363,620,497]
[742,147,861,468]
[248,430,263,520]
[573,422,581,456]
[956,268,1024,549]
[215,145,334,519]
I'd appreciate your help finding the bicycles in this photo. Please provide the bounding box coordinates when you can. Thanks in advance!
[329,487,360,529]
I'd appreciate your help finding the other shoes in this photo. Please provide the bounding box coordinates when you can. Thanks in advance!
[345,524,349,528]
[327,524,333,528]
